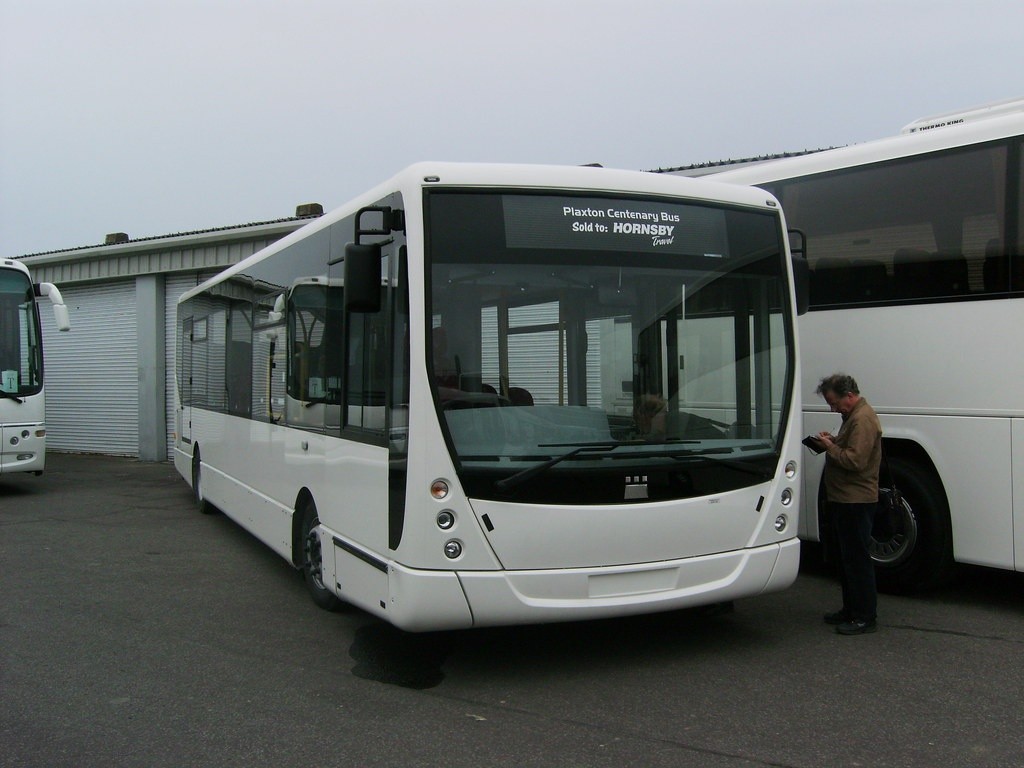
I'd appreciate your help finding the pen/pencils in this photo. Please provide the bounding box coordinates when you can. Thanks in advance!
[827,428,835,435]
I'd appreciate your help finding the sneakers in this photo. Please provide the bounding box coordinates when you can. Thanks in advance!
[836,618,879,635]
[823,610,850,623]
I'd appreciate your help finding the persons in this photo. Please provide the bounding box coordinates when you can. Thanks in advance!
[814,375,883,635]
[430,327,472,392]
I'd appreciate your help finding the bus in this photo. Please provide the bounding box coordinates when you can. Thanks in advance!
[601,100,1024,593]
[171,160,804,638]
[1,258,70,475]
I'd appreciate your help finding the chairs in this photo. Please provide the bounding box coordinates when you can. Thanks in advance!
[932,251,971,297]
[500,387,534,407]
[982,238,1008,294]
[815,257,851,304]
[842,259,888,302]
[892,248,933,300]
